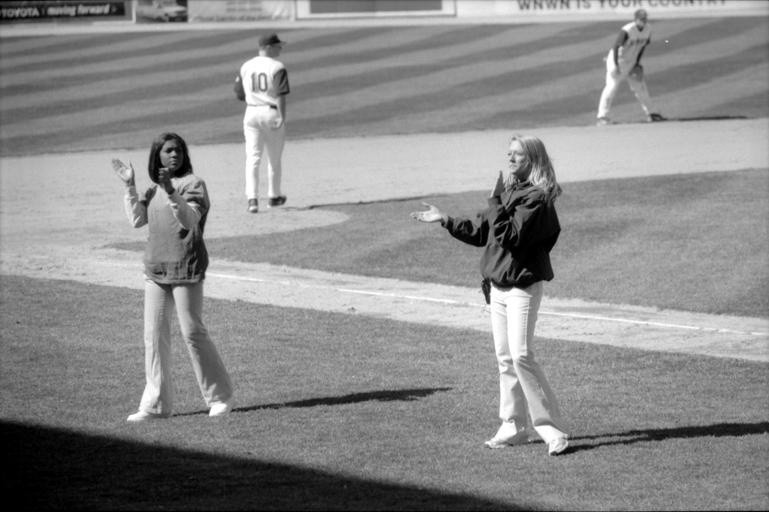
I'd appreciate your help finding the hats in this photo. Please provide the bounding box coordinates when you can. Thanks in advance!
[258,33,287,45]
[634,9,647,19]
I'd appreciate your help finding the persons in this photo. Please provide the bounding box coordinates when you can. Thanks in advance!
[233,34,292,214]
[409,133,571,458]
[110,132,236,422]
[593,7,661,124]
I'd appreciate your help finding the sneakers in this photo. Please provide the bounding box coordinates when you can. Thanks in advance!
[548,432,568,456]
[486,435,530,448]
[247,199,258,213]
[127,409,170,422]
[268,196,288,208]
[592,110,669,122]
[208,393,237,419]
